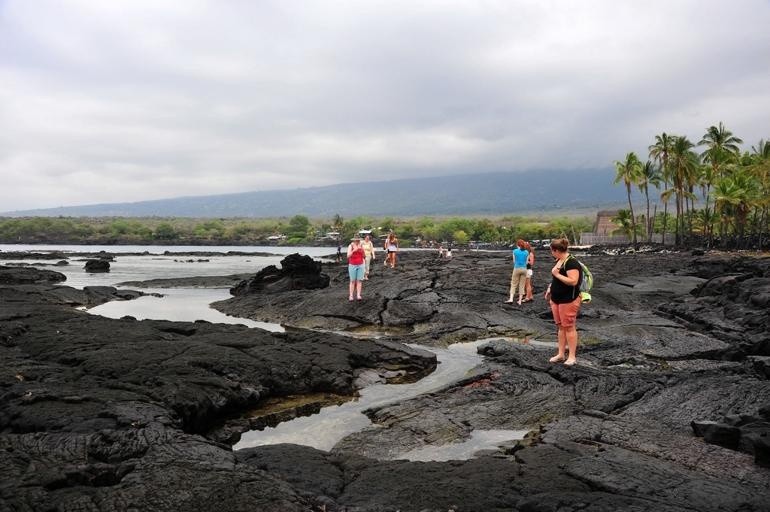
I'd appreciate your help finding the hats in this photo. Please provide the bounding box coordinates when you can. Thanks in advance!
[350,234,364,241]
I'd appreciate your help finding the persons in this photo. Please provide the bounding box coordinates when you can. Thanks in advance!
[523,242,536,303]
[336,244,344,262]
[542,237,584,366]
[387,231,398,269]
[503,238,530,306]
[361,234,375,281]
[444,247,453,259]
[346,234,366,302]
[436,244,445,258]
[384,233,390,267]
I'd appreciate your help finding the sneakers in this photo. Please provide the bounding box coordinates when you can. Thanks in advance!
[549,354,577,368]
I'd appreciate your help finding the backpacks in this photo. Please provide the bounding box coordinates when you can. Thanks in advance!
[563,254,594,294]
